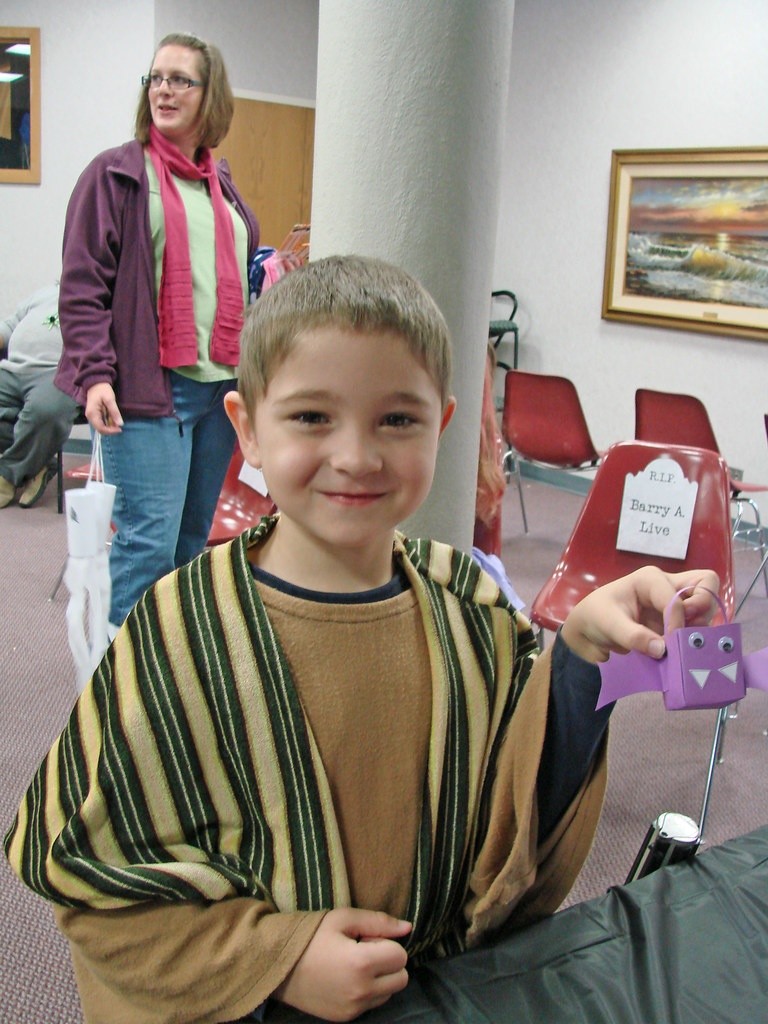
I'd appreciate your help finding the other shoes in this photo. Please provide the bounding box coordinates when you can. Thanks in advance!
[18,457,59,507]
[0,476,15,508]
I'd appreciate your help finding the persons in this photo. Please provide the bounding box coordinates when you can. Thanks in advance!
[0,277,81,508]
[55,35,258,645]
[2,253,720,1024]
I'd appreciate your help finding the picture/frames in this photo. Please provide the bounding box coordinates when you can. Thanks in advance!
[601,147,768,342]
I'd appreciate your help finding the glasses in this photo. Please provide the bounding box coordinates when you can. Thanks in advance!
[140,73,206,92]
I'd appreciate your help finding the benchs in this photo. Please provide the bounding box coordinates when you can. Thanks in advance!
[502,372,768,619]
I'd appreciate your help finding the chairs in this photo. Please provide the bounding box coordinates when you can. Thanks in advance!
[529,440,735,841]
[50,406,279,605]
